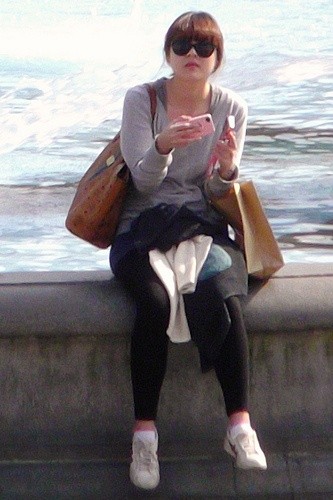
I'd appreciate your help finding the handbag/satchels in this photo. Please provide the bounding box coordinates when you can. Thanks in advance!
[131,201,179,255]
[213,180,285,280]
[65,126,131,249]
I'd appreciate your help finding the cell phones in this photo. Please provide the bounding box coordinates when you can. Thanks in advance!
[187,113,215,136]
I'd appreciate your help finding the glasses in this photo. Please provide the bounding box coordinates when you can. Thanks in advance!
[168,41,221,57]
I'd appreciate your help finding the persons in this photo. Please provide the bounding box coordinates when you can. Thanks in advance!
[108,10,269,491]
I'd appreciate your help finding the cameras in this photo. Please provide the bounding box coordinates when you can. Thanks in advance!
[220,115,235,141]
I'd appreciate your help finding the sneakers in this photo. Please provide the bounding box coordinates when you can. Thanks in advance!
[223,424,268,472]
[129,428,161,490]
